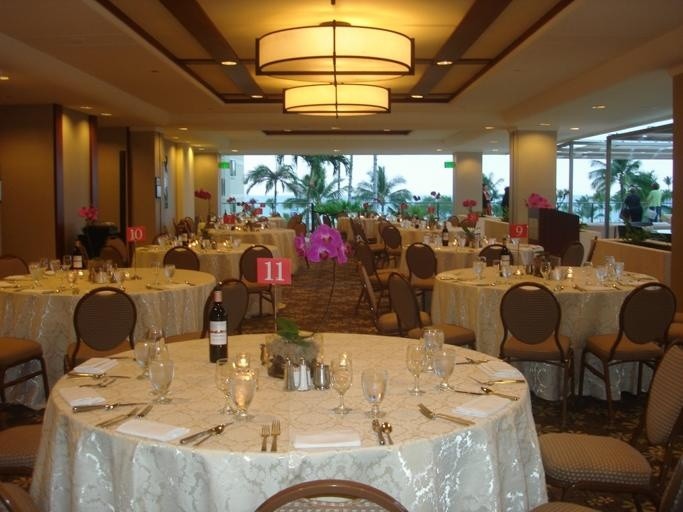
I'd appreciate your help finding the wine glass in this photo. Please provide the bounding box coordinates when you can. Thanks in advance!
[149,359,174,404]
[215,358,236,414]
[232,374,255,421]
[361,369,387,418]
[24,256,176,291]
[135,338,156,380]
[406,343,427,395]
[331,352,353,414]
[475,251,625,290]
[432,350,455,392]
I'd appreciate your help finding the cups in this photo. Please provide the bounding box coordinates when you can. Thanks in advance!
[234,353,251,373]
[420,327,444,373]
[147,326,168,355]
[148,343,170,396]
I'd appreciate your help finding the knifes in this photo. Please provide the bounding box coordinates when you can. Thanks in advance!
[86,352,134,364]
[71,402,149,413]
[65,367,135,389]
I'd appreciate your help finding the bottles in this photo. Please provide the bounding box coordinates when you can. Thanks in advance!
[440,218,450,249]
[284,355,296,391]
[179,218,192,248]
[208,283,232,366]
[295,351,310,391]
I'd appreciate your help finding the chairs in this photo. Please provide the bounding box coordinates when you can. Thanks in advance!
[258,479,410,512]
[159,278,249,337]
[161,245,201,271]
[62,234,135,271]
[349,219,442,320]
[532,442,683,512]
[385,271,477,350]
[2,255,28,277]
[534,339,683,511]
[288,217,310,270]
[1,337,51,511]
[491,281,578,414]
[61,287,138,378]
[354,262,432,338]
[581,281,679,426]
[233,244,281,335]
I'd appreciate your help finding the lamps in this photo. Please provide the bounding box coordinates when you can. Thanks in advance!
[255,20,415,83]
[282,84,391,117]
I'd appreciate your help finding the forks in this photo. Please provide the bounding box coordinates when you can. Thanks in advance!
[369,412,385,446]
[93,407,154,434]
[452,355,509,365]
[417,400,476,430]
[257,412,283,459]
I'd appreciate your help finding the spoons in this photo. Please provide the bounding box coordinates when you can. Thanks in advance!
[436,379,530,405]
[381,422,403,448]
[178,415,236,450]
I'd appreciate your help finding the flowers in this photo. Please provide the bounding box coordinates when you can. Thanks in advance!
[527,193,555,208]
[195,190,212,200]
[463,199,478,214]
[251,208,263,216]
[428,207,436,214]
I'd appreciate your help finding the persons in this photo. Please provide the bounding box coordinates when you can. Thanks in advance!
[483,184,494,215]
[501,187,509,208]
[646,182,662,222]
[624,186,643,222]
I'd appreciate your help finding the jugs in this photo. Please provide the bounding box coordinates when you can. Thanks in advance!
[313,363,331,390]
